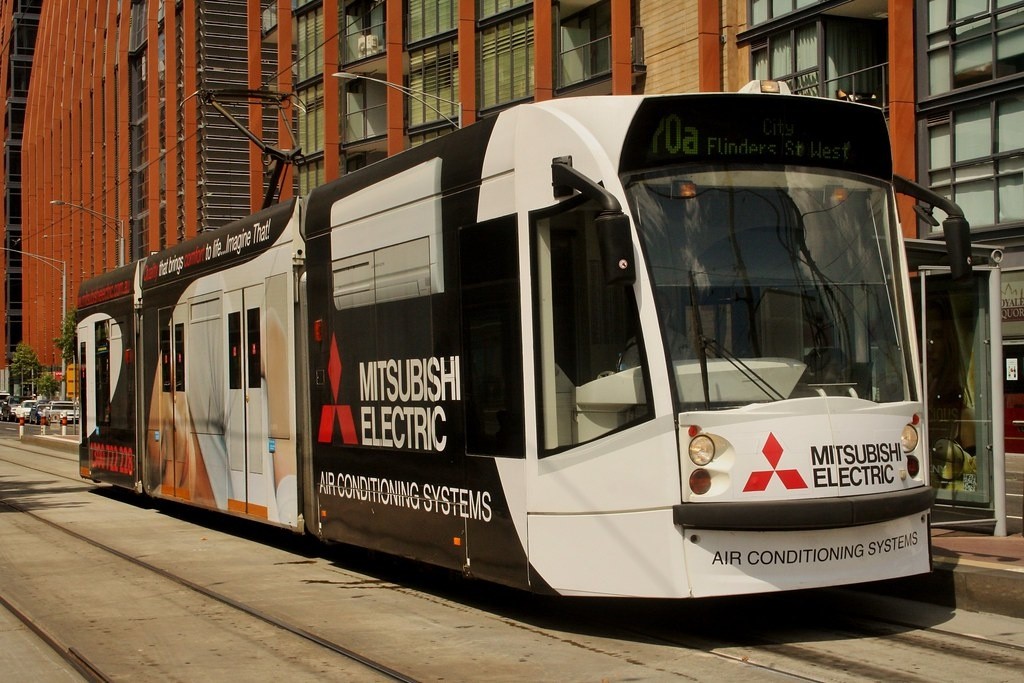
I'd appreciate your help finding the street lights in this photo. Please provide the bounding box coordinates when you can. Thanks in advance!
[49,200,125,270]
[330,73,464,131]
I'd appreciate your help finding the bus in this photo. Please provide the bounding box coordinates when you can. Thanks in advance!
[73,78,974,600]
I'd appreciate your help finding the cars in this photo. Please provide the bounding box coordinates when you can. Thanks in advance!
[0,392,80,425]
